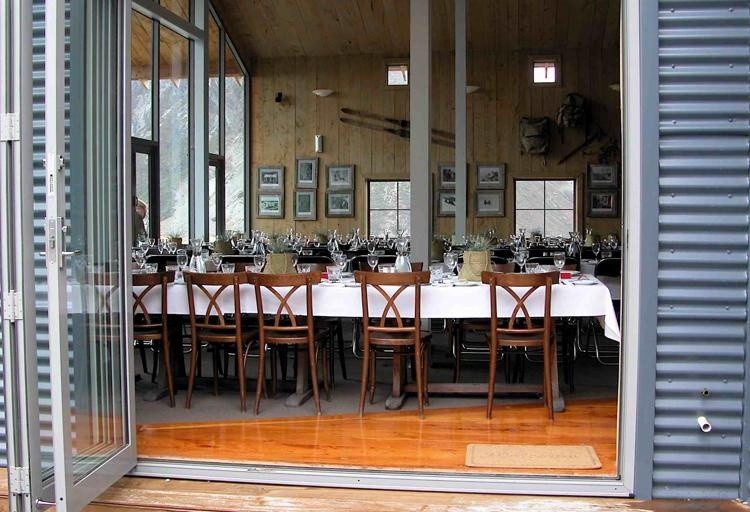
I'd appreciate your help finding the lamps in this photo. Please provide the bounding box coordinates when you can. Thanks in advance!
[312,88,334,98]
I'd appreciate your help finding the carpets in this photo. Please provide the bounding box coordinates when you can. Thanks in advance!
[465,443,601,469]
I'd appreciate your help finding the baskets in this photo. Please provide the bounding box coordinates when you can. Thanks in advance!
[584,235,601,247]
[316,235,328,244]
[459,250,494,282]
[213,239,233,254]
[262,253,297,274]
[168,238,181,249]
[431,239,441,259]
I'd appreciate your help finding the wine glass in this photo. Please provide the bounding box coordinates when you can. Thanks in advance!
[132,225,619,284]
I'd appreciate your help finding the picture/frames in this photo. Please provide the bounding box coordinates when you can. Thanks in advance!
[257,157,355,221]
[437,163,506,218]
[584,161,617,218]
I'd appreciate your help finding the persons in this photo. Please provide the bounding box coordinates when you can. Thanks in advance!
[136,199,149,221]
[135,197,147,247]
